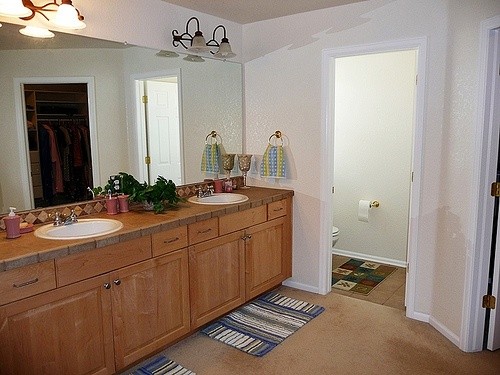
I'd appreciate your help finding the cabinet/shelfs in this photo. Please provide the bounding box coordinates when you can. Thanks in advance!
[21,75,94,206]
[189,199,296,335]
[1,221,187,375]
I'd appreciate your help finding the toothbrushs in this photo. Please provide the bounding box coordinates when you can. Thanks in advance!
[85,186,97,201]
[108,190,111,199]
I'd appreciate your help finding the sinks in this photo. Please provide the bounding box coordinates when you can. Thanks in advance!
[187,192,249,204]
[34,219,123,239]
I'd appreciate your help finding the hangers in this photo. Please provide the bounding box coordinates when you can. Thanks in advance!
[37,118,87,126]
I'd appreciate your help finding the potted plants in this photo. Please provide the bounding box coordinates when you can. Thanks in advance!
[138,178,185,216]
[93,173,152,215]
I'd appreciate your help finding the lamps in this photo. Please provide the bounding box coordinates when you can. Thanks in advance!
[171,16,237,60]
[0,0,87,32]
[156,50,178,58]
[19,25,55,37]
[184,55,205,62]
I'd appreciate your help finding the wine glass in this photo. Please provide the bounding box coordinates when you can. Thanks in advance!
[221,154,236,179]
[237,154,253,189]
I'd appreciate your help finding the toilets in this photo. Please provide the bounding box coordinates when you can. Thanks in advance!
[333,227,340,247]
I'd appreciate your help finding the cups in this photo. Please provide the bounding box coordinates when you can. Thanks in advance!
[118,195,130,213]
[105,197,119,214]
[213,180,225,193]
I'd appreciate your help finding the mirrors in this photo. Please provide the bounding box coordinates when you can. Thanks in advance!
[0,24,245,215]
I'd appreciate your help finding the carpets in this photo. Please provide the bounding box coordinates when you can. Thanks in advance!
[127,357,198,375]
[200,290,324,356]
[332,258,397,294]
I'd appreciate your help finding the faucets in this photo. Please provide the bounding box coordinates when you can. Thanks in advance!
[195,183,215,197]
[54,209,78,226]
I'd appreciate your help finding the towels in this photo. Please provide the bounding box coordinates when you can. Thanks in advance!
[260,144,286,177]
[200,143,220,172]
